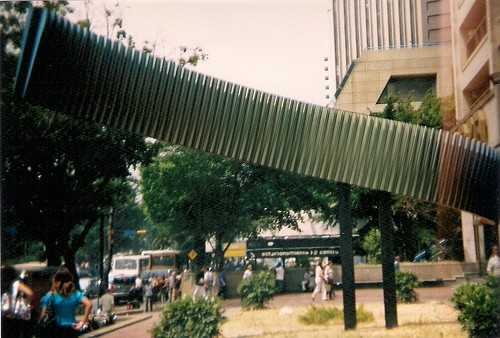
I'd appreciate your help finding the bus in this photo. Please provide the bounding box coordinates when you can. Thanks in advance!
[108,249,182,300]
[14,264,58,301]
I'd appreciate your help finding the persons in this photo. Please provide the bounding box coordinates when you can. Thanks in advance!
[486,244,500,275]
[192,265,213,298]
[127,266,181,312]
[269,262,285,294]
[242,264,253,278]
[216,268,229,301]
[98,289,114,325]
[393,256,400,270]
[438,250,449,261]
[1,265,93,338]
[301,261,337,301]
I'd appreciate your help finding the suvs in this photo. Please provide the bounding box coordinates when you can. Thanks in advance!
[108,274,137,305]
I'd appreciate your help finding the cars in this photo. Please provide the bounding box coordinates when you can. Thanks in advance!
[76,271,100,298]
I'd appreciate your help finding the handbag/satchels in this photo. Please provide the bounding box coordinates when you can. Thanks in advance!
[38,291,55,328]
[1,280,31,321]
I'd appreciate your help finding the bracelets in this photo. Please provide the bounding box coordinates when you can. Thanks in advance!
[80,321,84,327]
